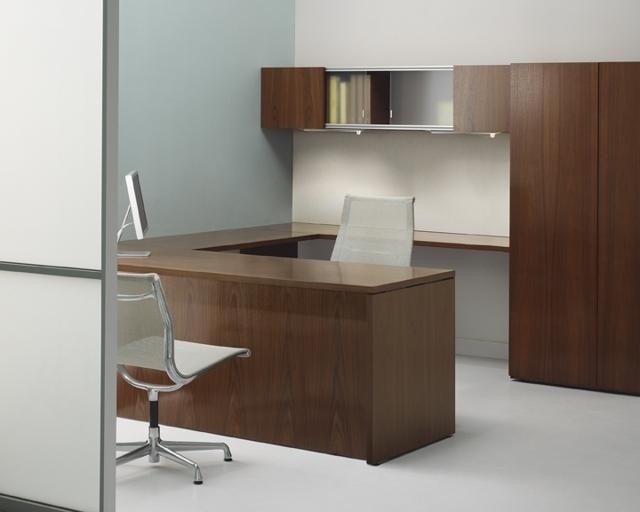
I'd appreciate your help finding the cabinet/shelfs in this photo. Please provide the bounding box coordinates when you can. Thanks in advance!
[257,64,509,135]
[509,55,638,397]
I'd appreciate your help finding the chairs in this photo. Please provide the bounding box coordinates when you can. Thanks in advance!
[328,193,416,269]
[111,270,254,486]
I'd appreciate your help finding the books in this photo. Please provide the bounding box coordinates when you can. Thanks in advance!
[328,72,390,125]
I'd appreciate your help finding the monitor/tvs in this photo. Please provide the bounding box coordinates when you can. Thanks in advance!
[117,168,153,256]
